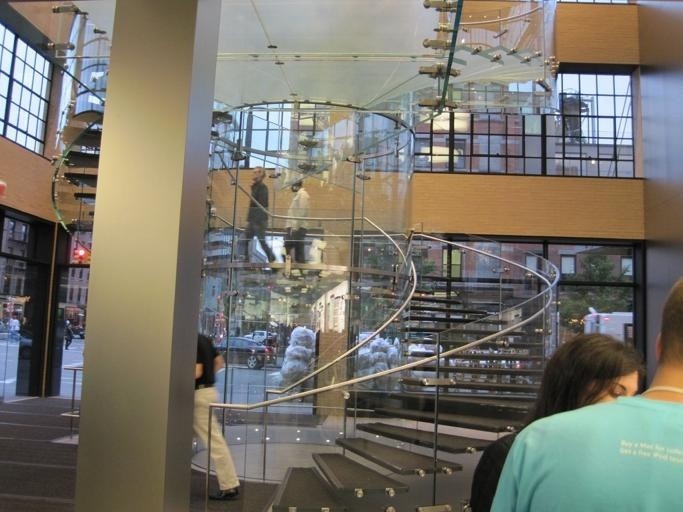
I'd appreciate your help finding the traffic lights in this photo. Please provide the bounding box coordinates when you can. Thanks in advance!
[74,246,86,260]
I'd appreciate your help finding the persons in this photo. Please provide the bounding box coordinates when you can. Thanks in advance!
[191,332,242,501]
[488,276,683,512]
[5,313,20,342]
[241,165,279,274]
[64,320,73,349]
[467,333,646,511]
[281,178,311,263]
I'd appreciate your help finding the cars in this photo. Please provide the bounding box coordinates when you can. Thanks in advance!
[215,336,276,369]
[248,330,272,343]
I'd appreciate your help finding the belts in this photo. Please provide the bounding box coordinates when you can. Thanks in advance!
[194,383,213,390]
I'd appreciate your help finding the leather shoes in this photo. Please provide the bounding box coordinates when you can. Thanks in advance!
[207,488,238,500]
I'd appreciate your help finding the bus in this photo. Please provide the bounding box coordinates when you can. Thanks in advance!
[583,311,634,343]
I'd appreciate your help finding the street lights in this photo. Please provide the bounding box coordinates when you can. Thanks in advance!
[491,266,510,330]
